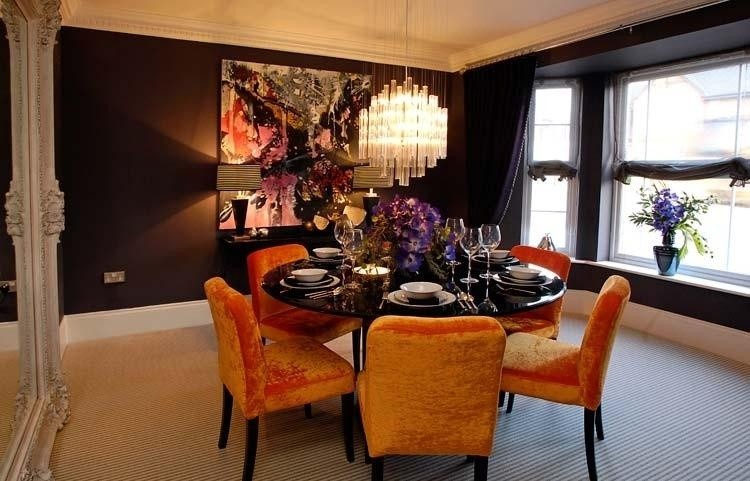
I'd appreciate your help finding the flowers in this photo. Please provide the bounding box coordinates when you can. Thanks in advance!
[634,180,721,259]
[351,195,458,283]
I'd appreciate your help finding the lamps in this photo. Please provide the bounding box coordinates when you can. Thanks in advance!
[216,163,263,240]
[352,0,450,189]
[350,165,393,225]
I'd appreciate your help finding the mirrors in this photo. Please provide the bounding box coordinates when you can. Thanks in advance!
[0,0,76,479]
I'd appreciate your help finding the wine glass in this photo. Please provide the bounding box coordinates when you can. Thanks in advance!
[475,279,498,314]
[478,222,502,280]
[342,226,365,291]
[459,226,483,283]
[333,218,355,270]
[443,217,466,267]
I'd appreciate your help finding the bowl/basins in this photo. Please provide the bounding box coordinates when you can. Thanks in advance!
[313,247,341,259]
[506,264,542,280]
[290,268,327,280]
[399,281,445,300]
[490,249,511,258]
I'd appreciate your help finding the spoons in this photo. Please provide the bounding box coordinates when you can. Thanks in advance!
[457,291,478,312]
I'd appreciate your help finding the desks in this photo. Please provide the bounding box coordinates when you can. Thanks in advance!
[254,250,567,467]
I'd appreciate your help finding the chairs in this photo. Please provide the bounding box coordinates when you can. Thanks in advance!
[246,243,359,421]
[492,244,570,408]
[356,313,506,480]
[495,272,632,480]
[201,274,354,480]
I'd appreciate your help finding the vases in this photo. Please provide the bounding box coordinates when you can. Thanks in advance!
[651,245,681,275]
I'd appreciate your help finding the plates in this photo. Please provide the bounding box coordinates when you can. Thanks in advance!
[471,255,520,264]
[308,254,347,263]
[493,272,553,286]
[279,274,342,290]
[386,290,457,308]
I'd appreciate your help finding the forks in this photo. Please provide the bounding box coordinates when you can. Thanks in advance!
[378,291,388,310]
[495,283,537,295]
[303,286,343,301]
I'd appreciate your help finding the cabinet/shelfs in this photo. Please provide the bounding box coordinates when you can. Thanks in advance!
[219,229,338,294]
